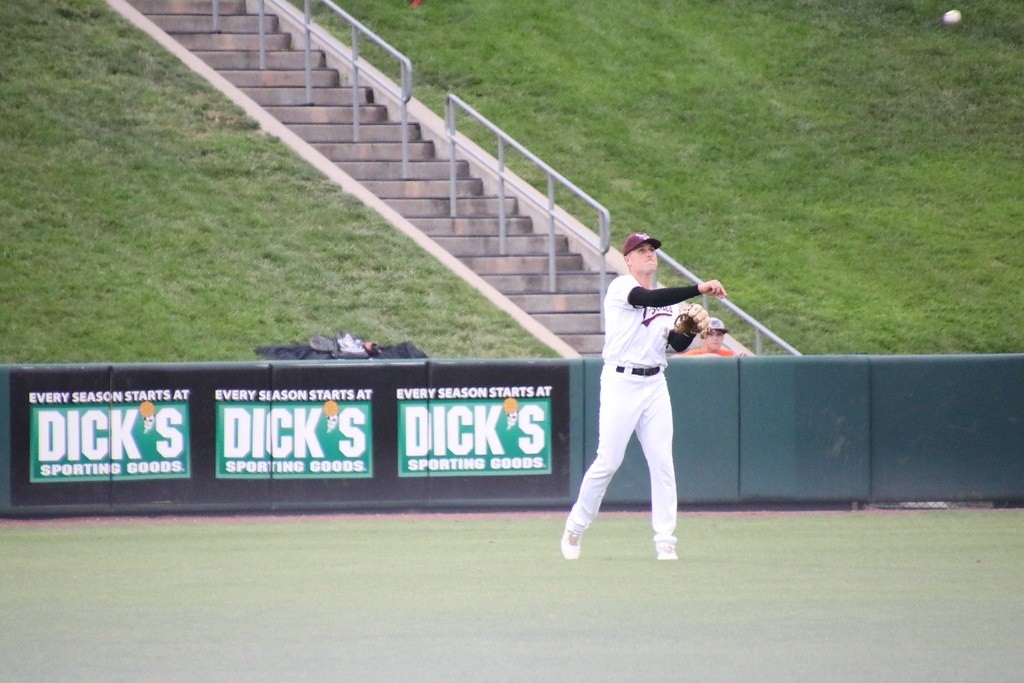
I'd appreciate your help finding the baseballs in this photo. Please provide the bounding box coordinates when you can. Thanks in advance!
[942,9,962,26]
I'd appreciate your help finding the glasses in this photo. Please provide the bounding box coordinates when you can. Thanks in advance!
[706,329,723,336]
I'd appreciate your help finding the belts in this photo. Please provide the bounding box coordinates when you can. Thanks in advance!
[616,366,660,376]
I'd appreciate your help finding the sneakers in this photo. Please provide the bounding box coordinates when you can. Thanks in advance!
[656,543,678,560]
[561,529,581,559]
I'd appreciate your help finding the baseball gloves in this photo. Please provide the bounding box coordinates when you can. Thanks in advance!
[673,302,710,339]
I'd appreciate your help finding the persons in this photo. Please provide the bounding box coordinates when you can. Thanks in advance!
[676,318,748,357]
[562,233,729,562]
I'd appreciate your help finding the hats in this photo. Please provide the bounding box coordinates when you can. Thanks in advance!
[623,232,661,256]
[701,317,728,338]
